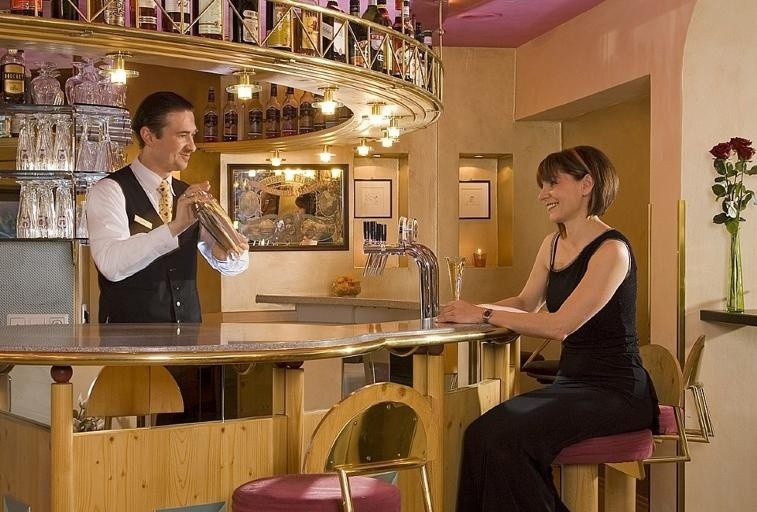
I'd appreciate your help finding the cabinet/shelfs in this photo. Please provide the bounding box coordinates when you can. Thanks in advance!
[0,103,131,242]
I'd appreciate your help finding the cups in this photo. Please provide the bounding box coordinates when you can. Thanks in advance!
[471,249,488,268]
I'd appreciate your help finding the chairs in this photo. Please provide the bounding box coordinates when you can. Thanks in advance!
[229,380,440,511]
[83,366,184,429]
[520,334,716,511]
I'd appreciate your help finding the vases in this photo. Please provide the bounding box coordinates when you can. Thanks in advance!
[725,229,744,314]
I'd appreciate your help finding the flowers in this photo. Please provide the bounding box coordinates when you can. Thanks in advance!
[708,137,756,312]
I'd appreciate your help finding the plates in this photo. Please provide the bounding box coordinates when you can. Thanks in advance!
[238,186,340,241]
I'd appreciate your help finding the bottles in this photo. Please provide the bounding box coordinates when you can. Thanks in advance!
[261,84,352,137]
[222,92,238,141]
[202,87,218,143]
[247,81,263,139]
[190,191,244,253]
[6,0,435,95]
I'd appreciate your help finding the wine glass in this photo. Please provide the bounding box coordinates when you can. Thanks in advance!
[0,50,137,111]
[445,255,466,300]
[11,112,129,242]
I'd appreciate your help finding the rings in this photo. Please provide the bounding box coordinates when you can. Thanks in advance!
[182,192,186,198]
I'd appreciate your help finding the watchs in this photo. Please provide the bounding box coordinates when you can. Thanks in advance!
[481,308,495,324]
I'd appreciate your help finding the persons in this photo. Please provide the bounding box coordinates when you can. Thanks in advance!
[430,145,660,510]
[85,90,250,322]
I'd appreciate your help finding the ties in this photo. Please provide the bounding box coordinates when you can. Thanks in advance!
[157,180,173,224]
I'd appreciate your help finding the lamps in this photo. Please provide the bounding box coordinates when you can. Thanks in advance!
[97,51,141,87]
[224,67,403,168]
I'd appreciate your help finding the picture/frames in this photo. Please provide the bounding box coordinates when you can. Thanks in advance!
[226,163,393,253]
[459,180,491,220]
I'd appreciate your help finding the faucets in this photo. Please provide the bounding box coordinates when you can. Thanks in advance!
[360,215,441,322]
